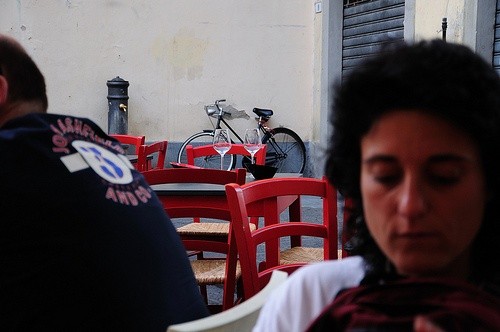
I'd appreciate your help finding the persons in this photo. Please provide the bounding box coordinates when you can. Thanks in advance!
[0,35,208,332]
[253,41,500,331]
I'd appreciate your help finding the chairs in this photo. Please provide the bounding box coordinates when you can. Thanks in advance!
[110,135,353,332]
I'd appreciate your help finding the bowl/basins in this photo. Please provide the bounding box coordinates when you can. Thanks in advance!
[245,163,279,180]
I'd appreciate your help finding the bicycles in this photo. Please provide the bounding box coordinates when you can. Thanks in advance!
[177,98,306,174]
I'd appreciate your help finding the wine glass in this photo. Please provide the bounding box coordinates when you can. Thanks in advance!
[213,129,231,170]
[243,128,262,179]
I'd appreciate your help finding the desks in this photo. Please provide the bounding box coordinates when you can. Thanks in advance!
[147,172,303,297]
